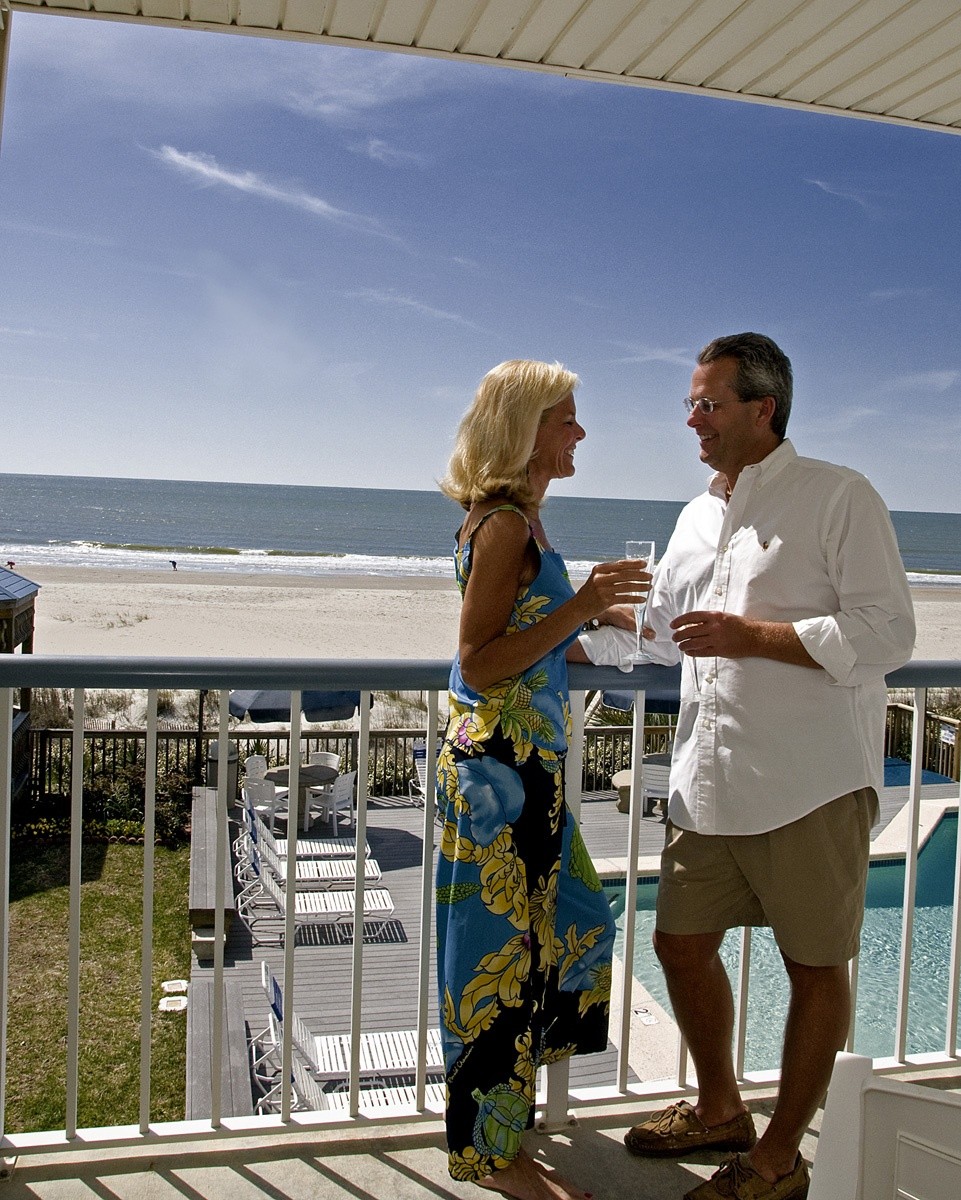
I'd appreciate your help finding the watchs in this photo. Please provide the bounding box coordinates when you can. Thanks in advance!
[589,617,600,630]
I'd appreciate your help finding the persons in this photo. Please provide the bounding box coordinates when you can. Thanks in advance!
[169,560,177,571]
[432,358,655,1200]
[5,561,15,569]
[567,332,917,1200]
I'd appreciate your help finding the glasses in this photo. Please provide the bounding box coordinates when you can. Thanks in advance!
[683,394,768,414]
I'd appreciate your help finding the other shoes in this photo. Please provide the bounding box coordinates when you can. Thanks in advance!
[684,1149,811,1200]
[625,1101,758,1158]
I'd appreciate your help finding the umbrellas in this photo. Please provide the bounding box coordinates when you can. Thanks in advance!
[229,689,374,723]
[601,689,680,714]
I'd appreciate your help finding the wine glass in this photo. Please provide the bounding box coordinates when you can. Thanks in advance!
[625,541,658,662]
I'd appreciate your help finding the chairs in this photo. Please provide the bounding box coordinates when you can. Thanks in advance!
[233,742,446,1115]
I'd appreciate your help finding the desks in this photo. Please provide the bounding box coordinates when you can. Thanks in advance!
[264,764,339,828]
